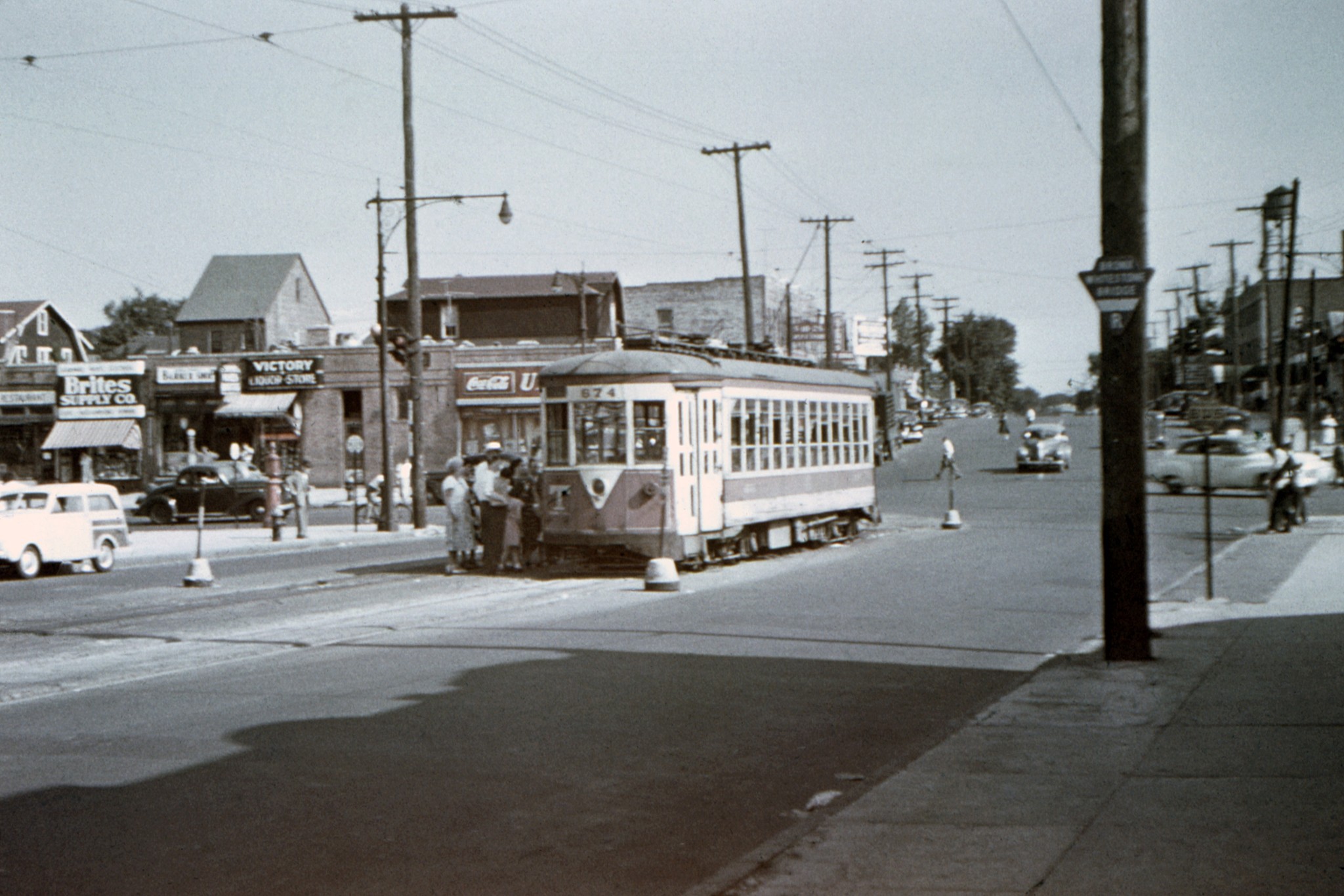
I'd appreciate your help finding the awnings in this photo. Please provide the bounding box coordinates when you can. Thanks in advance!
[39,418,143,450]
[215,391,297,417]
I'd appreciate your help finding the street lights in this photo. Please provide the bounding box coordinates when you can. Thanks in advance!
[377,178,514,533]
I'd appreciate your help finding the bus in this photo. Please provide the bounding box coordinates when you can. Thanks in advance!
[534,323,882,573]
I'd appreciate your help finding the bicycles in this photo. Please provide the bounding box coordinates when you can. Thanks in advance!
[357,504,415,525]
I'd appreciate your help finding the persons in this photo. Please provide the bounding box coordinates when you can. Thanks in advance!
[1266,436,1310,535]
[439,442,545,574]
[937,436,963,478]
[999,409,1010,433]
[80,451,94,483]
[286,460,312,538]
[366,474,384,523]
[1026,407,1036,424]
[397,457,413,502]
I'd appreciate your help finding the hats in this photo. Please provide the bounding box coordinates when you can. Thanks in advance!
[485,442,502,454]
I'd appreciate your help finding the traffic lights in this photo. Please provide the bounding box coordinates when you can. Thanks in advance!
[388,332,419,362]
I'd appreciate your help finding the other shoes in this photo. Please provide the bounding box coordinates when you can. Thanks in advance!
[514,567,527,574]
[445,563,468,573]
[461,559,479,571]
[496,566,510,575]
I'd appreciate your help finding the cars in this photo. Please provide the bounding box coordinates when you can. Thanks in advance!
[1016,424,1073,473]
[0,481,130,579]
[873,398,993,464]
[1144,434,1337,493]
[426,452,519,505]
[1145,390,1252,433]
[130,460,297,526]
